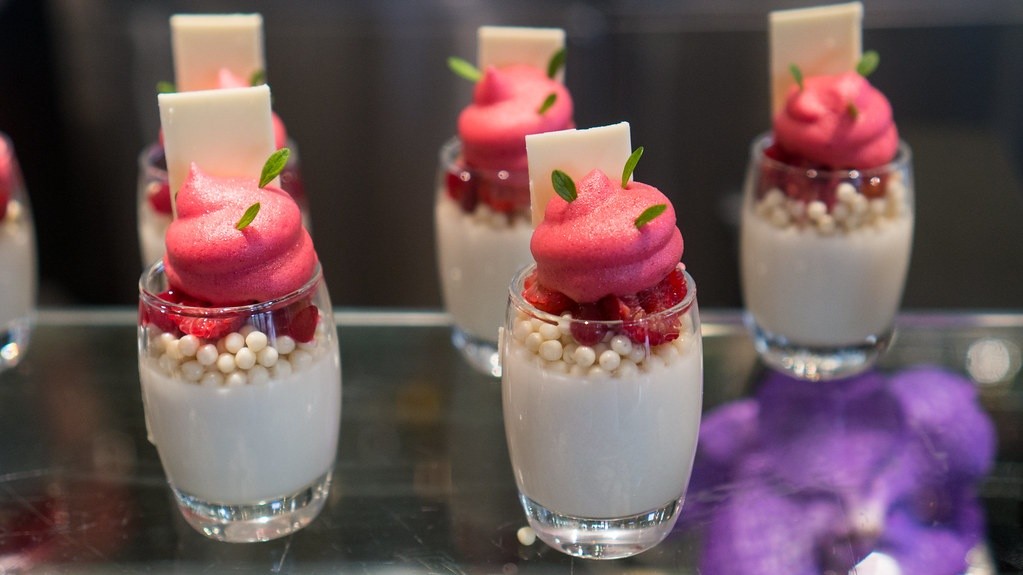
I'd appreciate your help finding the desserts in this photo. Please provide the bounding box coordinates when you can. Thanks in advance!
[741,1,915,345]
[501,121,704,519]
[138,12,341,506]
[435,24,575,347]
[0,134,34,343]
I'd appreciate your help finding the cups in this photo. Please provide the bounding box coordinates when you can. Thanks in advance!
[434,135,536,380]
[0,138,35,371]
[137,256,342,544]
[137,137,309,269]
[501,261,702,560]
[740,132,915,382]
